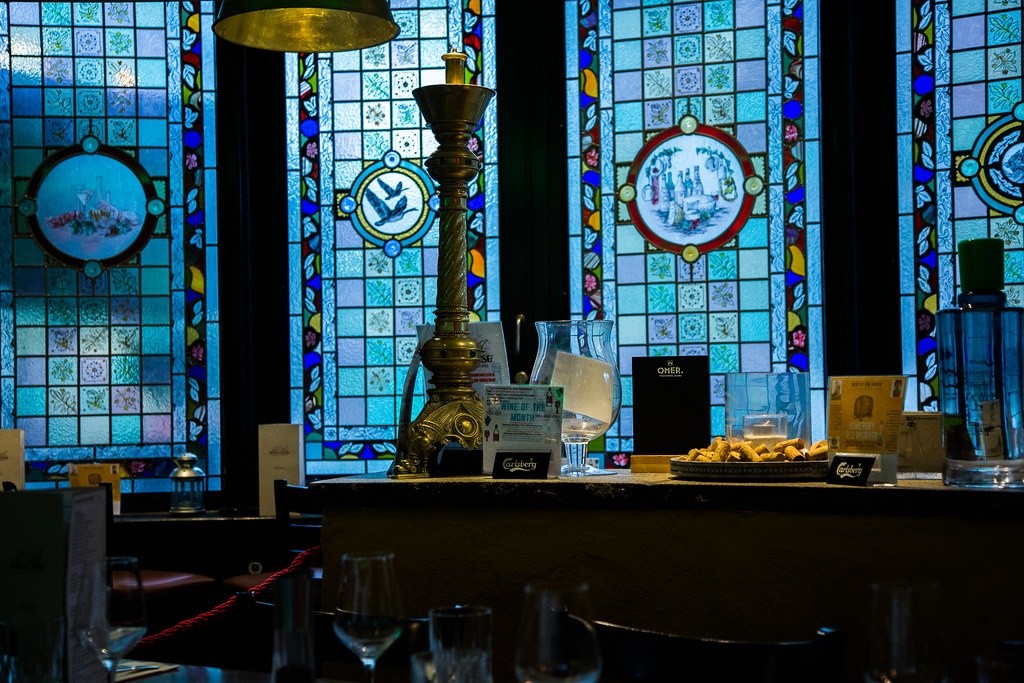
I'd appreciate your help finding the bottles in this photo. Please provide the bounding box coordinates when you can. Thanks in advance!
[169,453,207,516]
[934,238,1024,489]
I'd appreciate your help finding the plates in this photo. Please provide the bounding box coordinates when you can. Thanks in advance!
[669,456,829,480]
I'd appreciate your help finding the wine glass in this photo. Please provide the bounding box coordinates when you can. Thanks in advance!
[73,557,148,683]
[529,320,623,477]
[332,551,403,683]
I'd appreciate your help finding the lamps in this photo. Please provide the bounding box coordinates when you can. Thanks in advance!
[212,0,400,52]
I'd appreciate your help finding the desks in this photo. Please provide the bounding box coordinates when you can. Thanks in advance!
[110,504,323,579]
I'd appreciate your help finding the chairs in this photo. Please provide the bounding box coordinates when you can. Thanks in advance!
[100,482,214,601]
[595,616,850,683]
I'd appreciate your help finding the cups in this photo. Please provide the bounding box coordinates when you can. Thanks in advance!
[742,415,787,448]
[515,584,602,683]
[428,605,493,683]
[751,425,775,435]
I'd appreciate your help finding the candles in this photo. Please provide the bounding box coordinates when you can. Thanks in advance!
[442,49,468,84]
